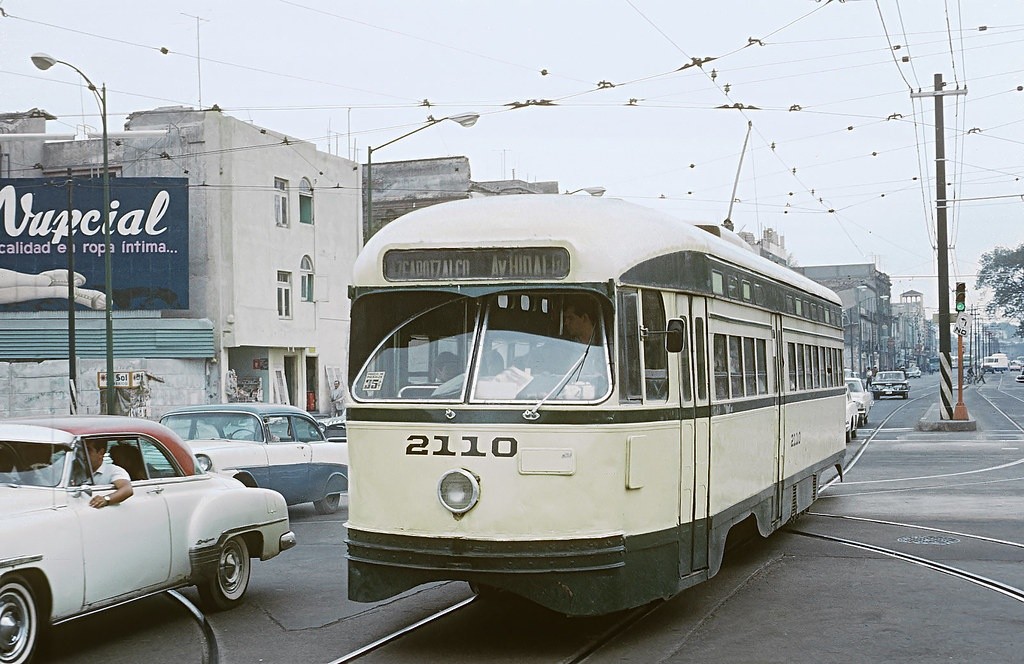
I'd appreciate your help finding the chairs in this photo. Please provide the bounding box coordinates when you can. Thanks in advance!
[884,373,892,379]
[23,444,52,470]
[848,383,859,392]
[895,373,902,379]
[108,445,148,481]
[182,424,220,439]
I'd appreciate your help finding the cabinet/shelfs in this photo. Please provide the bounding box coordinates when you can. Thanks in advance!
[237,376,263,402]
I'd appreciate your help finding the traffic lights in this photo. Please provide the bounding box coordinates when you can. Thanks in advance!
[956,282,965,312]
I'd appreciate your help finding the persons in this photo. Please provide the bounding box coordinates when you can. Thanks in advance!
[434,349,460,382]
[873,364,878,377]
[32,439,134,508]
[515,301,608,380]
[0,268,113,311]
[226,415,280,442]
[967,366,975,377]
[866,367,873,391]
[848,383,858,392]
[330,380,344,417]
[976,367,987,383]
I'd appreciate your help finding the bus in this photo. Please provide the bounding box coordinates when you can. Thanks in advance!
[342,192,848,621]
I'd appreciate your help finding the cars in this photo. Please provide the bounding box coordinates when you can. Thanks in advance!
[309,410,346,443]
[872,370,911,399]
[844,369,873,443]
[904,354,1024,378]
[158,402,347,513]
[1,418,295,664]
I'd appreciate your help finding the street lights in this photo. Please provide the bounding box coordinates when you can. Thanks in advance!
[366,111,478,238]
[31,51,119,416]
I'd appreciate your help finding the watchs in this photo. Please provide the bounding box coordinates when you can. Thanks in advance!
[103,495,111,506]
